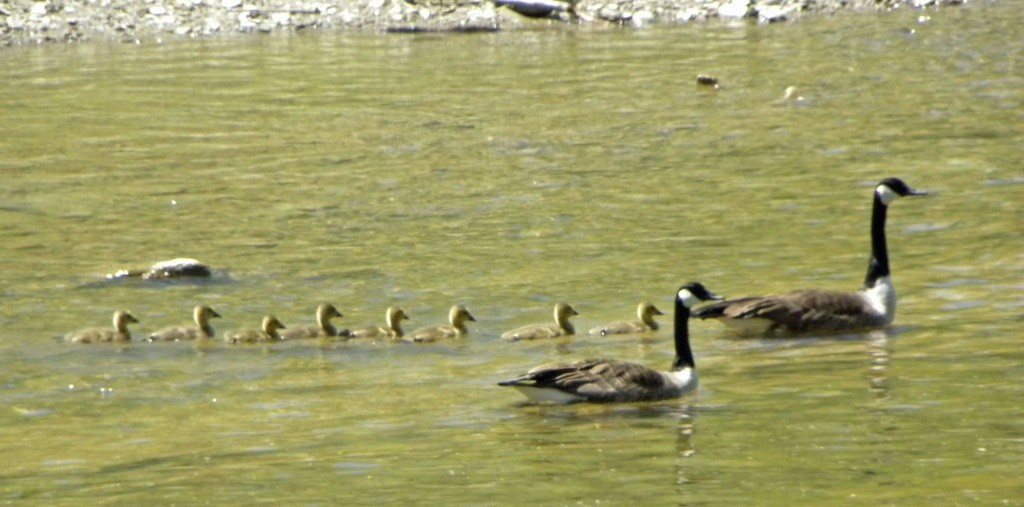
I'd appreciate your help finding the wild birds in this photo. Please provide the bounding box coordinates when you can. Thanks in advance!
[279,303,342,340]
[690,179,928,338]
[223,314,288,343]
[141,304,222,341]
[59,309,141,344]
[339,305,410,342]
[496,279,726,403]
[585,302,665,335]
[401,305,477,344]
[501,301,578,342]
